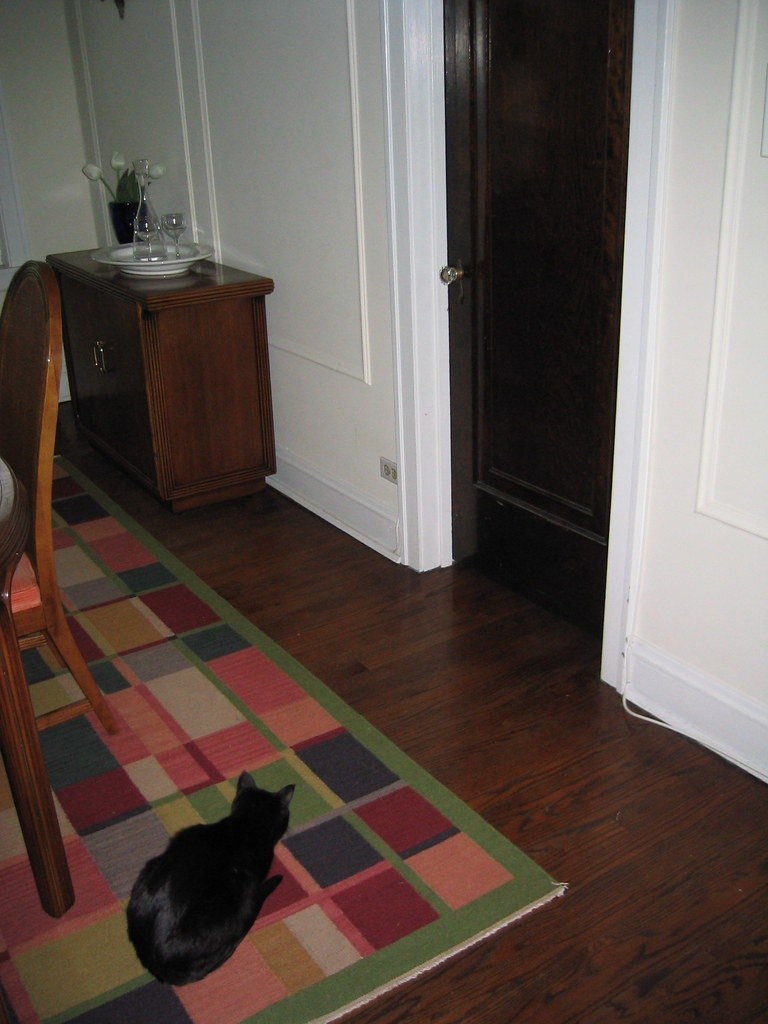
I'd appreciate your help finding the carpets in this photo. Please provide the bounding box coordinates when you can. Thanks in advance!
[0,451,567,1024]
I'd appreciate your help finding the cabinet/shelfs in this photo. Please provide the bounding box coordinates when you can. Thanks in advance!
[46,245,274,517]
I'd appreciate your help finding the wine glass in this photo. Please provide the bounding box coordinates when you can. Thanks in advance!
[134,216,161,261]
[161,211,186,259]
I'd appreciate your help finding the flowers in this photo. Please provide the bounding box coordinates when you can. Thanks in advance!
[81,150,167,201]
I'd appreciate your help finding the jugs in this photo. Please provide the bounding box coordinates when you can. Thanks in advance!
[131,157,169,262]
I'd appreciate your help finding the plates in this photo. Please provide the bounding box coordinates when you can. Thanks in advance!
[91,240,214,275]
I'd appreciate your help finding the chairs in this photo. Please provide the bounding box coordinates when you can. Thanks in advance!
[0,252,126,751]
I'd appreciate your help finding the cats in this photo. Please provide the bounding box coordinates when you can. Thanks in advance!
[126,769,295,986]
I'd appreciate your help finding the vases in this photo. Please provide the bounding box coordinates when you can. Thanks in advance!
[108,200,151,244]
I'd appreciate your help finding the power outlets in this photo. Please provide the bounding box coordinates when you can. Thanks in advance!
[379,457,399,484]
